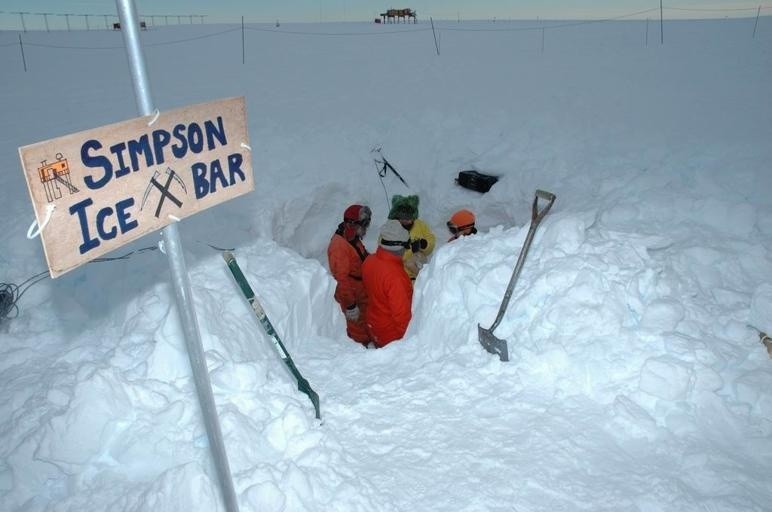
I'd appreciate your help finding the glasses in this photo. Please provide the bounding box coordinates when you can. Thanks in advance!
[447,222,458,234]
[361,219,370,227]
[404,238,410,249]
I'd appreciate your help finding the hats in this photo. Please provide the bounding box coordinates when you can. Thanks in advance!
[388,195,419,220]
[341,205,370,241]
[380,220,410,251]
[451,210,475,230]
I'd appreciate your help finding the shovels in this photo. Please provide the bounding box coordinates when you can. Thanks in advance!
[477,188,556,363]
[209,244,320,422]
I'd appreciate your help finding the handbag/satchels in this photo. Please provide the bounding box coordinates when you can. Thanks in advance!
[459,171,497,193]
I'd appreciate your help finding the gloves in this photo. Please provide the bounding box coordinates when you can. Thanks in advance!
[346,306,360,321]
[420,239,427,249]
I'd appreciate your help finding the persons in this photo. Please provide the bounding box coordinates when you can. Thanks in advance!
[362,220,413,349]
[328,204,371,346]
[388,195,436,281]
[447,209,477,242]
